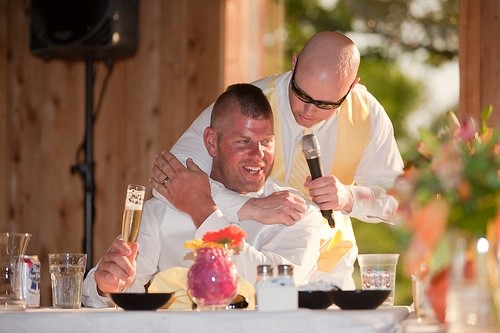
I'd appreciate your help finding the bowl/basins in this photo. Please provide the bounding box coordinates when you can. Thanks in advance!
[329,291,390,309]
[299,291,337,311]
[110,292,172,310]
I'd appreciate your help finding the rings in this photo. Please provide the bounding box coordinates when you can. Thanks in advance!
[162,177,170,184]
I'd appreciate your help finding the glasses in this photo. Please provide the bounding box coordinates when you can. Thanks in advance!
[289,54,354,111]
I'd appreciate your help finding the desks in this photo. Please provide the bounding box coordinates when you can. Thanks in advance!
[0,306,415,333]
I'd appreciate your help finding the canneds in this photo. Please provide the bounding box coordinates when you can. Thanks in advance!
[9,254,40,308]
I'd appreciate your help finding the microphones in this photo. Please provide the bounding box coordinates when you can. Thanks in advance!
[301,134,335,228]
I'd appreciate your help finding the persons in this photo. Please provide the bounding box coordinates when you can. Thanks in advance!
[79,83,329,310]
[152,30,407,289]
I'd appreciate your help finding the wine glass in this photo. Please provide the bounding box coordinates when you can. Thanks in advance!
[114,184,146,310]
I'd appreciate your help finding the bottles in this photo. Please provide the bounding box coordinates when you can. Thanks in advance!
[276,265,298,310]
[254,264,276,310]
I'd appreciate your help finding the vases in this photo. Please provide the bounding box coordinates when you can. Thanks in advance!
[414,224,500,333]
[187,248,240,310]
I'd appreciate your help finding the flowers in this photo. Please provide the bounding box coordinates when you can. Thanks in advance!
[386,106,500,234]
[183,226,247,255]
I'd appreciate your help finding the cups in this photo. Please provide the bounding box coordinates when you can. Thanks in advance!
[0,232,32,312]
[357,254,399,308]
[48,253,87,309]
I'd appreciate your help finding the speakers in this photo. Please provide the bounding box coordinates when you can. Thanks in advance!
[27,0,140,62]
